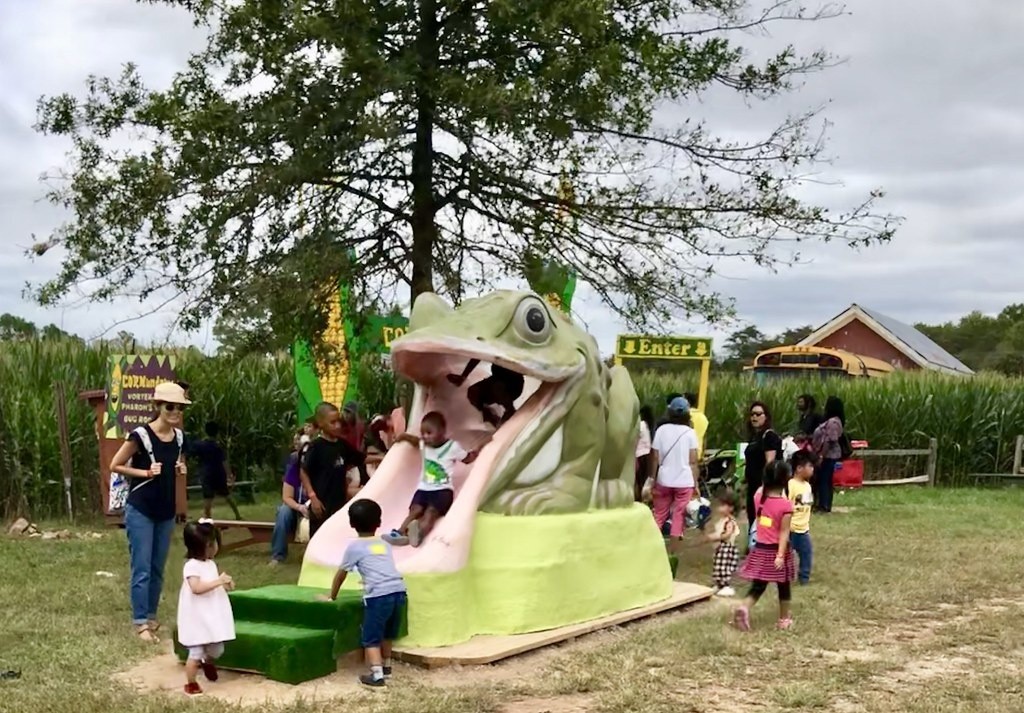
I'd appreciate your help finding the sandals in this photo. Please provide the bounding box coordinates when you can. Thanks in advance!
[139,628,152,641]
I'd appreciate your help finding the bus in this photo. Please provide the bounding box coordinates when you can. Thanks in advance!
[741,344,900,381]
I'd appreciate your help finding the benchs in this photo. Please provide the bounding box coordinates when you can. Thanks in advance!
[187,481,258,504]
[212,520,297,553]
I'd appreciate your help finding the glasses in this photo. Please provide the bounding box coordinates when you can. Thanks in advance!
[750,411,765,416]
[166,404,184,411]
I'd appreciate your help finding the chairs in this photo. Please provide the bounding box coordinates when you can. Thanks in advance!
[699,447,738,498]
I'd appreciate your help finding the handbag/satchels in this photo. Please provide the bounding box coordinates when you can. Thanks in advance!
[686,489,711,529]
[293,517,310,544]
[838,433,854,458]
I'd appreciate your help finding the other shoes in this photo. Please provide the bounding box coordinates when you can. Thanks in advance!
[496,408,515,429]
[718,586,735,597]
[184,683,203,697]
[775,619,793,630]
[382,528,409,546]
[200,660,219,681]
[268,559,280,566]
[735,607,750,631]
[383,666,393,678]
[407,519,422,547]
[359,674,387,690]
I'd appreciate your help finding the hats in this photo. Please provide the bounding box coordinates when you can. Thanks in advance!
[153,382,192,404]
[344,401,356,414]
[667,397,690,413]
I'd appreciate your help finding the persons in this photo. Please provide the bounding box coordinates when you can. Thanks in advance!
[634,391,845,630]
[196,421,245,520]
[177,521,236,694]
[317,497,408,691]
[446,358,524,430]
[267,385,407,568]
[109,382,189,643]
[380,411,492,547]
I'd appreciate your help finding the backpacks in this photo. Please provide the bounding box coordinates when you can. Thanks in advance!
[108,426,182,513]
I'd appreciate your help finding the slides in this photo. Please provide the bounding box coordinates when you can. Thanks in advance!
[307,383,570,565]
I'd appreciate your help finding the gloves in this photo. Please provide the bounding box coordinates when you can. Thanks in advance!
[642,477,654,502]
